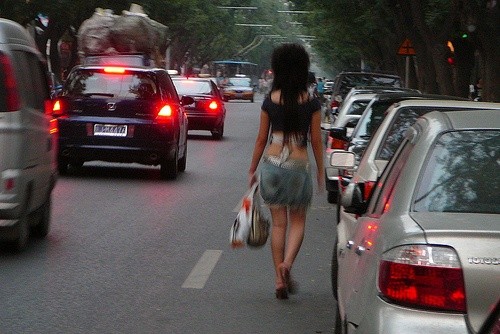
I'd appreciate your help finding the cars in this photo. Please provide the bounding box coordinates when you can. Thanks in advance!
[46,72,66,97]
[170,78,228,139]
[313,70,500,334]
[197,74,220,91]
[222,74,257,103]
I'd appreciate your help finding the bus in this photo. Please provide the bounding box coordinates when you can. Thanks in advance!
[210,61,259,93]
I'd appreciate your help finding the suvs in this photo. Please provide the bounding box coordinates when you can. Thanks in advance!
[53,64,194,182]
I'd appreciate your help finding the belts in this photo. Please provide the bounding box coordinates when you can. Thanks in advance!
[263,156,310,170]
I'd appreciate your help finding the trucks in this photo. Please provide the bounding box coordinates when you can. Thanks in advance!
[79,50,158,69]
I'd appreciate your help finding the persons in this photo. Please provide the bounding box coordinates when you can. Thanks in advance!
[248,43,324,300]
[316,77,325,99]
[307,72,320,101]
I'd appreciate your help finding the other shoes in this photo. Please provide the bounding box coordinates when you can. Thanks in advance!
[275,283,287,300]
[279,263,292,294]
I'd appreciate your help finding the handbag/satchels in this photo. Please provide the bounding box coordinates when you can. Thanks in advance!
[230,179,260,248]
[247,185,270,246]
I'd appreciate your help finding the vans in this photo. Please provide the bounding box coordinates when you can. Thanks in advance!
[0,17,75,254]
